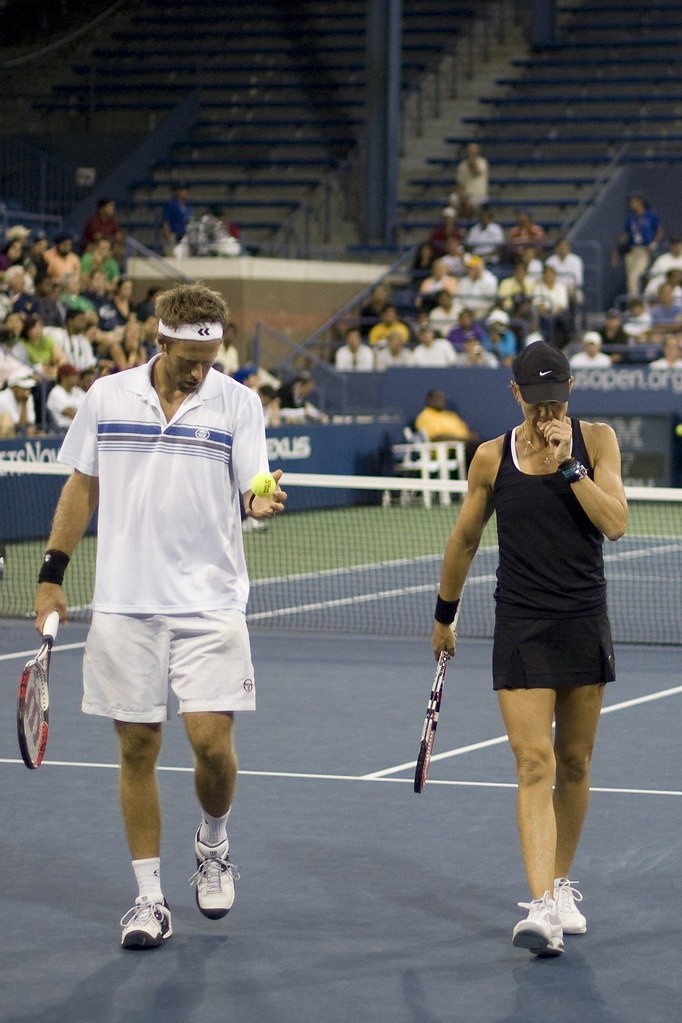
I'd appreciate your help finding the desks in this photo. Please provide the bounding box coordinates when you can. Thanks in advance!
[374,440,469,510]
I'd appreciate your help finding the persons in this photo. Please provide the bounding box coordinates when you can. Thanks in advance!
[415,388,492,500]
[334,189,682,375]
[33,285,288,949]
[432,342,629,957]
[454,141,488,217]
[0,180,315,438]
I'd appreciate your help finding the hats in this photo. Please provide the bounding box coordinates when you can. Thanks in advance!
[513,340,570,403]
[8,370,36,389]
[582,330,600,345]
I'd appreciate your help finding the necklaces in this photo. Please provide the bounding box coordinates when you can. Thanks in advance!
[155,366,185,404]
[524,431,556,464]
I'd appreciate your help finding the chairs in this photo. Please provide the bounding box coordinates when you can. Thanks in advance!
[402,427,469,505]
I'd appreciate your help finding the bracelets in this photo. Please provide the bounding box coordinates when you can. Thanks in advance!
[562,461,581,480]
[249,493,256,511]
[434,594,460,623]
[558,457,576,471]
[36,549,70,585]
[565,464,587,484]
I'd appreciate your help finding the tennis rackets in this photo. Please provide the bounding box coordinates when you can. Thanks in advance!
[16,610,62,771]
[412,579,469,797]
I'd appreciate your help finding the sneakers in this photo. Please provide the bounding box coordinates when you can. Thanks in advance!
[510,892,566,956]
[121,899,172,948]
[551,877,586,935]
[193,822,239,919]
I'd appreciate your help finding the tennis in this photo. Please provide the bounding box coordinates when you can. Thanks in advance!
[251,473,277,497]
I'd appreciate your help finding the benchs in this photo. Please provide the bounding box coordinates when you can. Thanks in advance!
[0,0,682,372]
